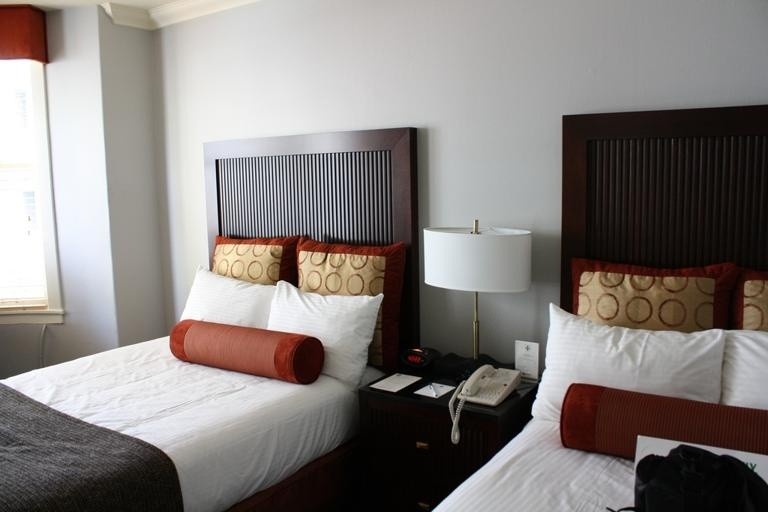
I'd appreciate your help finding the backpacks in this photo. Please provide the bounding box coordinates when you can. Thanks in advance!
[604,445,768,512]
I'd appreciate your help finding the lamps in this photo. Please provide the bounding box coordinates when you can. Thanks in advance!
[419,216,536,378]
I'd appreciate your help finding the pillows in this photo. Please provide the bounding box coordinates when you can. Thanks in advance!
[295,235,407,375]
[570,258,738,333]
[736,267,768,333]
[178,264,275,335]
[559,382,768,462]
[210,234,299,289]
[169,319,325,386]
[722,330,768,414]
[266,277,384,386]
[531,301,728,428]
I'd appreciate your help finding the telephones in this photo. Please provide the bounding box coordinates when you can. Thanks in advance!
[449,364,521,444]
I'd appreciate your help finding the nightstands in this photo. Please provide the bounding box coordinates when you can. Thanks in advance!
[357,358,542,478]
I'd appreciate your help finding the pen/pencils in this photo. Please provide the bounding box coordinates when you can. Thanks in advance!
[429,383,439,397]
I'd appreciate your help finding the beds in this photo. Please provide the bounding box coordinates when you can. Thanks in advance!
[419,103,767,510]
[0,124,420,512]
